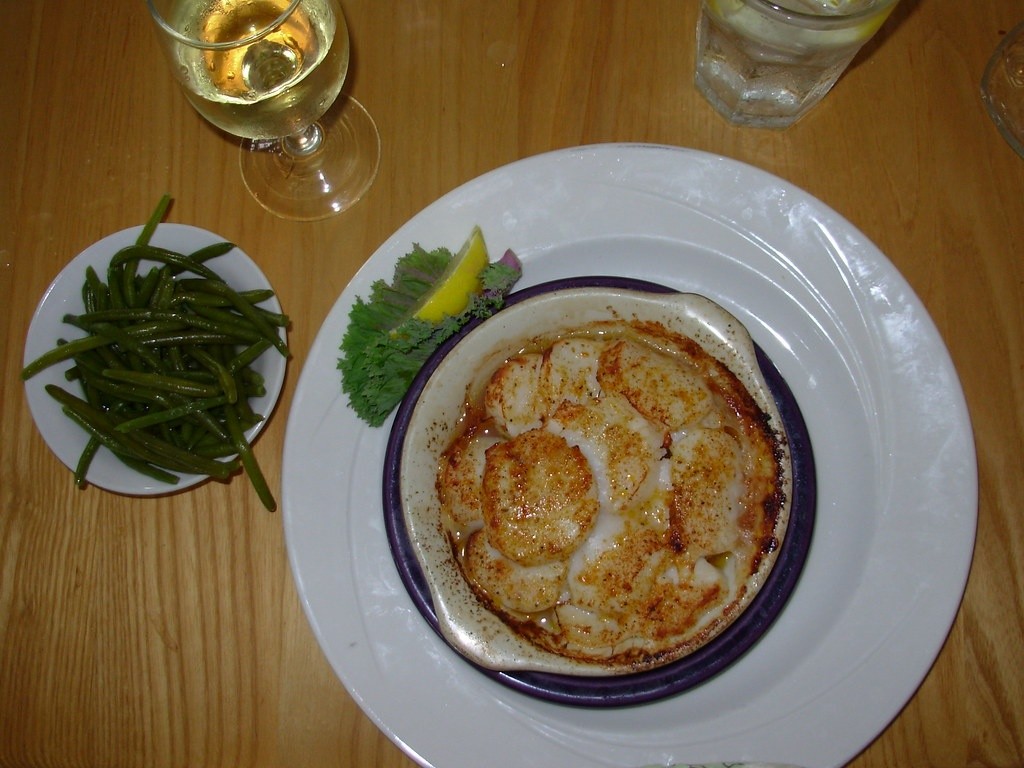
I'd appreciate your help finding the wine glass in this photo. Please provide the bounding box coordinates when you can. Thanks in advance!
[149,0,380,221]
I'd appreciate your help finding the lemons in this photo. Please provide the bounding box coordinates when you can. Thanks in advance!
[386,226,489,338]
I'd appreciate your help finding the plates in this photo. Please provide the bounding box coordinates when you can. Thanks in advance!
[24,224,287,495]
[383,276,817,709]
[282,144,977,768]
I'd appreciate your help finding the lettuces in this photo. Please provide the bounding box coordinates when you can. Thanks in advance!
[339,248,520,425]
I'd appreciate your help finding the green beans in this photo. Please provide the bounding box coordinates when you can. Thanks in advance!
[20,196,291,511]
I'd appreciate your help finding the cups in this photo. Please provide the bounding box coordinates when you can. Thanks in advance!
[694,0,898,128]
